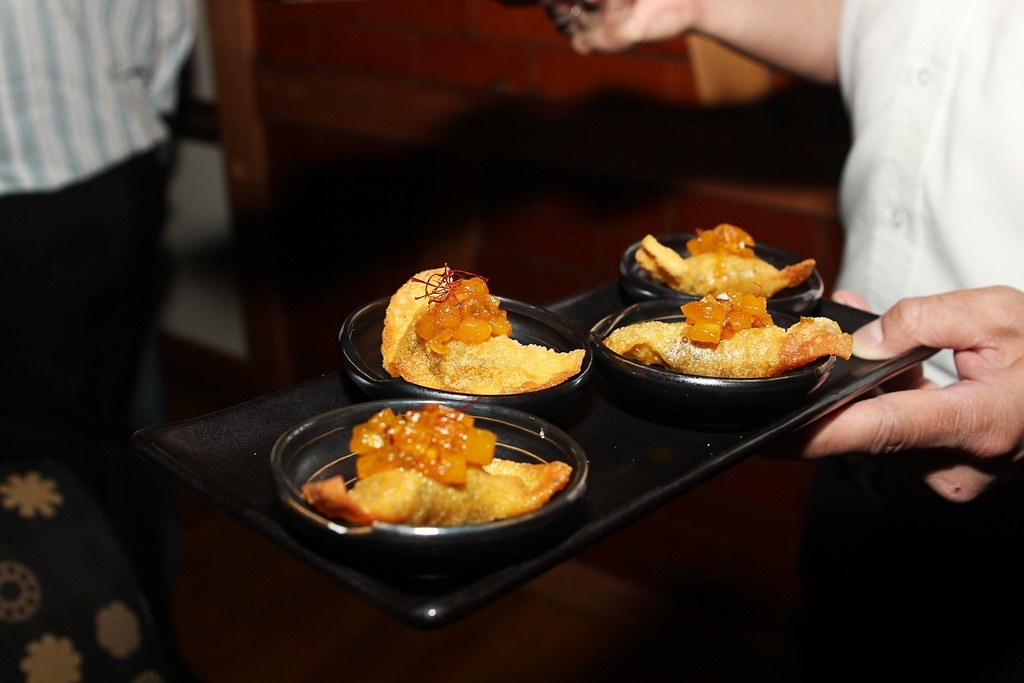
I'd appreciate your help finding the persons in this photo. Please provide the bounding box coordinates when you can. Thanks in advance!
[548,0,1023,682]
[1,0,200,613]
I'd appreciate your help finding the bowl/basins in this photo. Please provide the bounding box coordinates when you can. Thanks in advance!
[336,295,594,412]
[618,234,824,317]
[269,400,591,550]
[587,298,837,428]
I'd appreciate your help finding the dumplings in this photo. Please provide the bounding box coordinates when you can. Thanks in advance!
[300,456,573,525]
[631,234,820,297]
[604,316,854,378]
[382,268,586,395]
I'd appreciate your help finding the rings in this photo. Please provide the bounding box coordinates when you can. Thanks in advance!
[555,5,580,27]
